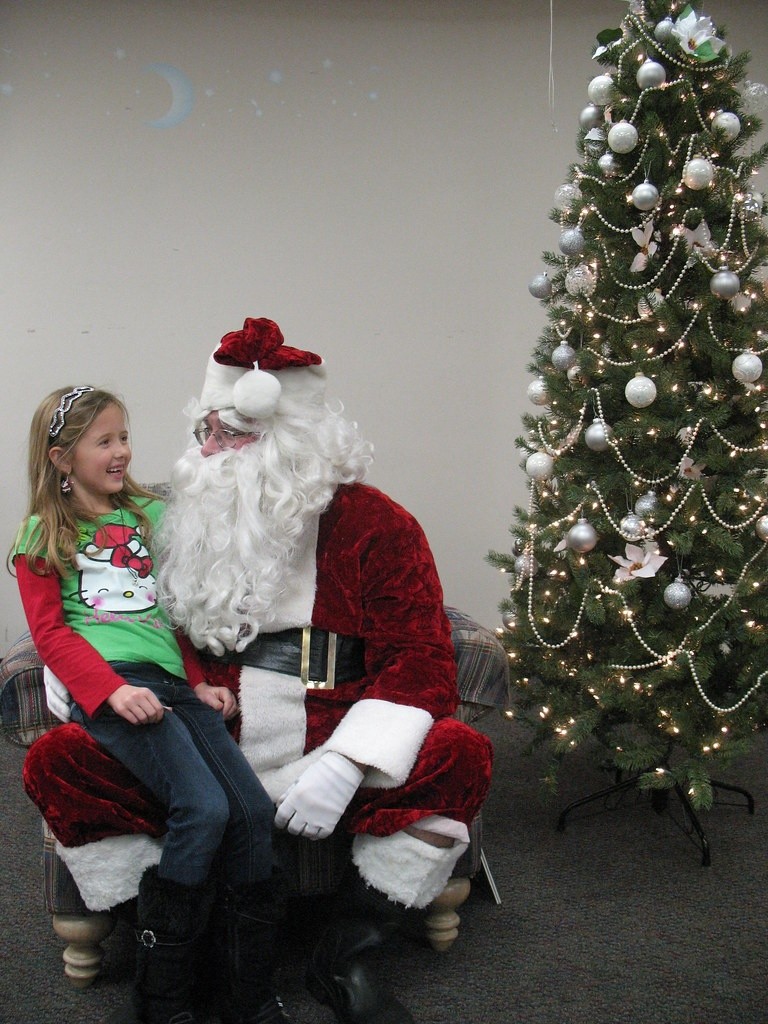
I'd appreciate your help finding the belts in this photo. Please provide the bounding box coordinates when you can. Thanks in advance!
[196,624,368,691]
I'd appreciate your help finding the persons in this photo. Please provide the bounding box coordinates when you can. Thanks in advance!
[20,316,495,1024]
[6,387,291,1024]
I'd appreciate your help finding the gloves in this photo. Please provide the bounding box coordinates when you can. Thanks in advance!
[274,751,364,841]
[42,665,70,724]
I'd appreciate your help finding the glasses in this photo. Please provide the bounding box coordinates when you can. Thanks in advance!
[192,427,254,449]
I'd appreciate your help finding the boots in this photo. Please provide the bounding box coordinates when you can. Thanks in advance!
[224,883,279,1024]
[134,866,218,1024]
[308,870,416,1024]
[103,896,183,1024]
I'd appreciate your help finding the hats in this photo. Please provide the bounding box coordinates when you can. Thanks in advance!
[196,316,328,421]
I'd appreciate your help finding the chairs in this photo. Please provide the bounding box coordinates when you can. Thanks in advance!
[0,603,510,989]
